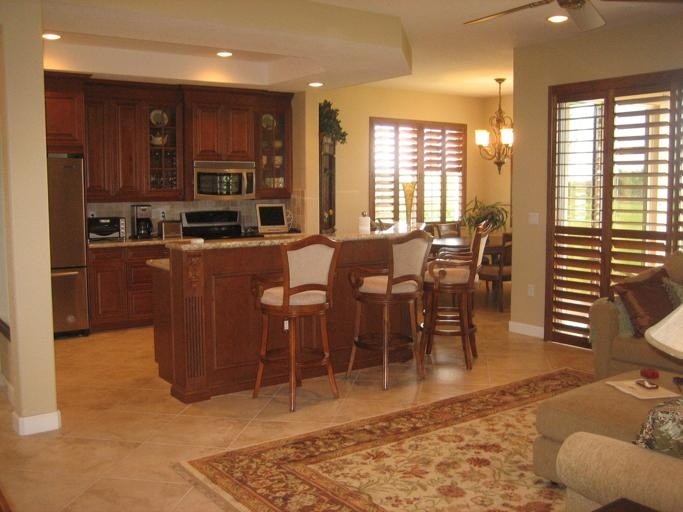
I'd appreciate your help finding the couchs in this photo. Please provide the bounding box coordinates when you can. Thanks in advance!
[587,249,683,383]
[531,370,683,487]
[555,430,683,511]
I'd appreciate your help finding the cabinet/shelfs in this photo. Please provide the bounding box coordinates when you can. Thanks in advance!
[88,243,153,328]
[42,68,92,156]
[85,77,294,205]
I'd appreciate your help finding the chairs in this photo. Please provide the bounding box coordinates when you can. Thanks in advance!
[344,229,435,392]
[418,214,480,372]
[433,223,461,239]
[252,233,344,414]
[479,231,512,314]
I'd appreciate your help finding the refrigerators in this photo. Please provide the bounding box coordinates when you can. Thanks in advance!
[47,157,90,340]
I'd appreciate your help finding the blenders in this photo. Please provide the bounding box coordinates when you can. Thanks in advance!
[131,204,153,242]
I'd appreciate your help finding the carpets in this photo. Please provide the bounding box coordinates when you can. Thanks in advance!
[169,365,595,511]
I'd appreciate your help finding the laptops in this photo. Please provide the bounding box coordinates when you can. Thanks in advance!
[254,202,304,238]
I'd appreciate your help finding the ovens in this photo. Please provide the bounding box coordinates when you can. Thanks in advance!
[194,161,256,201]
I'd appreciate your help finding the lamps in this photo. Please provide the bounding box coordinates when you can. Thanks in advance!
[474,76,513,175]
[547,15,570,25]
[644,302,683,396]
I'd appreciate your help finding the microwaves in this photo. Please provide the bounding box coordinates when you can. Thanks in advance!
[87,216,126,241]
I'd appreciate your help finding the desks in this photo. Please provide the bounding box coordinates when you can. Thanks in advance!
[429,233,512,309]
